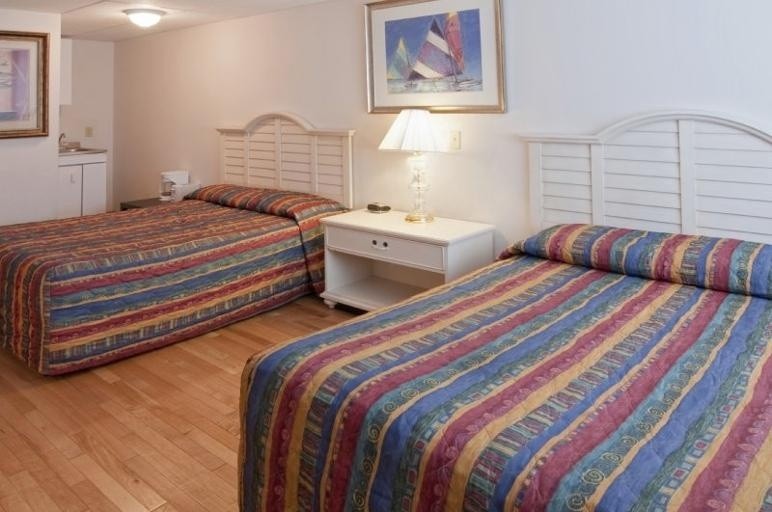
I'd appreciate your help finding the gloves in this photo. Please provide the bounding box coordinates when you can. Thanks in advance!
[387,12,483,90]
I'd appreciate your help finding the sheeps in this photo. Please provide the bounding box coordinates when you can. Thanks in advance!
[59,132,66,142]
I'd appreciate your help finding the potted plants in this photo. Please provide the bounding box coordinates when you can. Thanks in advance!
[0,29,49,138]
[364,1,507,114]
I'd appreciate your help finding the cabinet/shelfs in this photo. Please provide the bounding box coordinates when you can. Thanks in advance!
[169,188,176,204]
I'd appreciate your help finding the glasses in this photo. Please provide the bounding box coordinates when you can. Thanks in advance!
[123,7,166,30]
[374,104,459,224]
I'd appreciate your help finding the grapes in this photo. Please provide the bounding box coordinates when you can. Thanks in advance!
[314,209,495,313]
[118,198,172,211]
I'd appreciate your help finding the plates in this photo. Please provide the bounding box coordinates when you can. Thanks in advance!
[57,145,109,220]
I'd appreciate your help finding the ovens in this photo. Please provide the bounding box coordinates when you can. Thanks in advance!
[0,112,357,377]
[236,111,772,512]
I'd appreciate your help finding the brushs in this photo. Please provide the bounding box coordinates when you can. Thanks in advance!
[158,171,188,202]
